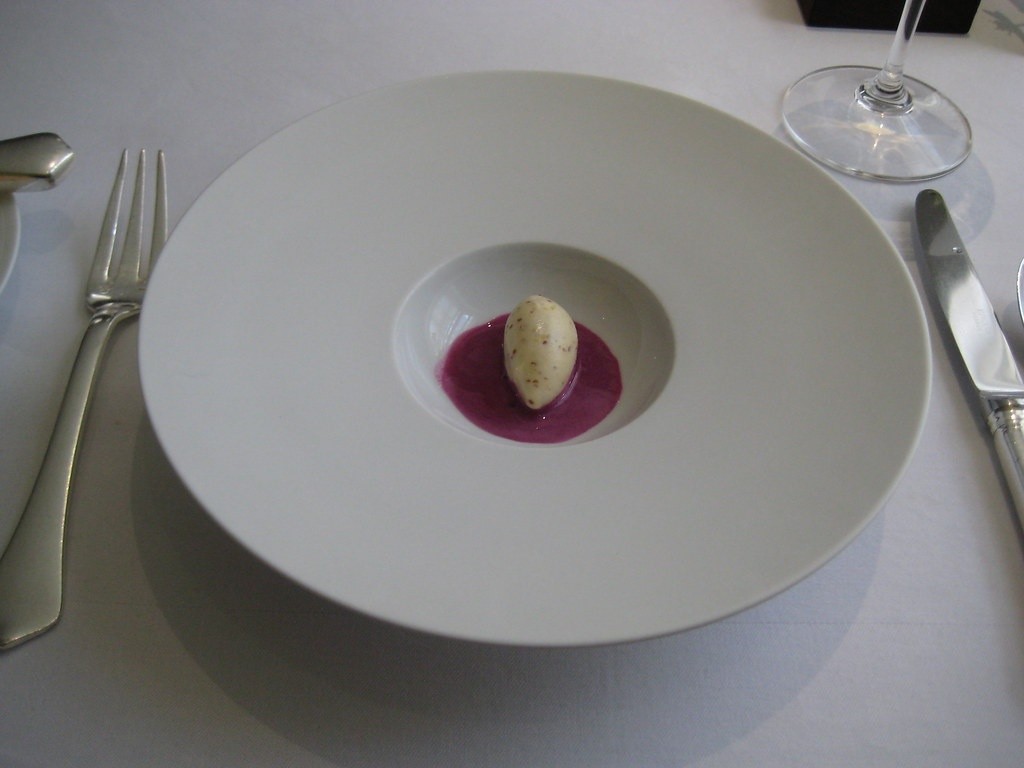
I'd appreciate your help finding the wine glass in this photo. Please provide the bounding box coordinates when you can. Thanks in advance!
[779,0,971,185]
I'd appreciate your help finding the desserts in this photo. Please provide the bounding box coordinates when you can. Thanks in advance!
[503,293,579,409]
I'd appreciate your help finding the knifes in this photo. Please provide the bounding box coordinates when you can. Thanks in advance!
[913,187,1024,538]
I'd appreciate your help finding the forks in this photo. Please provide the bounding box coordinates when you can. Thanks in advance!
[0,146,168,652]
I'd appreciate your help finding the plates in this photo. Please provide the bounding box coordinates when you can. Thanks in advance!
[135,68,933,651]
[0,191,21,288]
